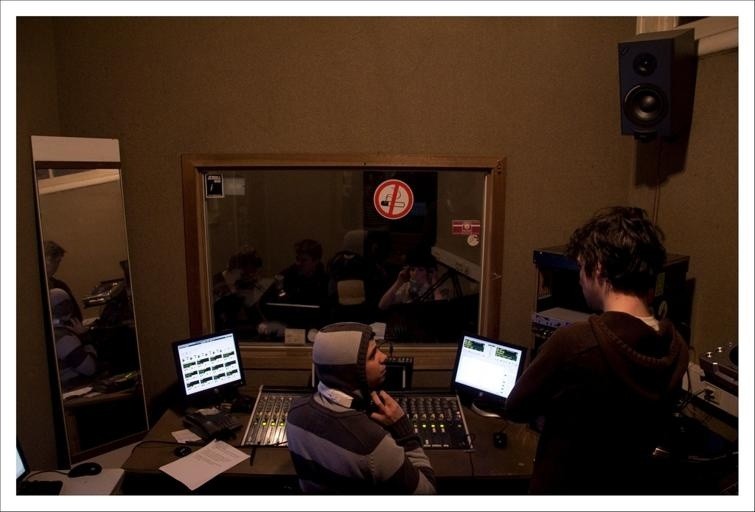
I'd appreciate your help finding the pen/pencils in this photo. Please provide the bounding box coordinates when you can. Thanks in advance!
[250,445,258,466]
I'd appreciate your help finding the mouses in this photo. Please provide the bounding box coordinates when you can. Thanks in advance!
[493,431,507,450]
[174,446,192,457]
[68,462,102,478]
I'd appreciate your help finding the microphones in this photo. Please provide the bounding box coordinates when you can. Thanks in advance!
[408,286,421,303]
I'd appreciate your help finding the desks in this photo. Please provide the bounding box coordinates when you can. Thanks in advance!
[123,371,544,475]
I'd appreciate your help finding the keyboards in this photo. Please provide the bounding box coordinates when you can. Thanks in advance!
[20,480,63,495]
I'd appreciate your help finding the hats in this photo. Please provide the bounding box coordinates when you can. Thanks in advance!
[312,321,372,410]
[50,288,72,316]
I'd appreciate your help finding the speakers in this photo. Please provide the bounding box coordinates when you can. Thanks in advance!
[617,27,696,136]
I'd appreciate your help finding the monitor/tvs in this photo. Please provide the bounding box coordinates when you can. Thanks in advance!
[170,329,247,406]
[16,437,31,481]
[450,332,528,418]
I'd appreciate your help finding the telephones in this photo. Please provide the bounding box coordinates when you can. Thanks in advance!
[183,407,242,441]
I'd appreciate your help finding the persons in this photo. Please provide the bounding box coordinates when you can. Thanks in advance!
[378,251,452,310]
[50,287,100,392]
[214,239,328,334]
[44,240,83,326]
[290,321,440,495]
[504,206,690,495]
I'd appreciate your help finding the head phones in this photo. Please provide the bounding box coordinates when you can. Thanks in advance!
[53,314,76,326]
[316,381,385,414]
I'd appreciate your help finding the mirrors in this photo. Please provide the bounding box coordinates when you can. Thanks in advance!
[29,135,148,466]
